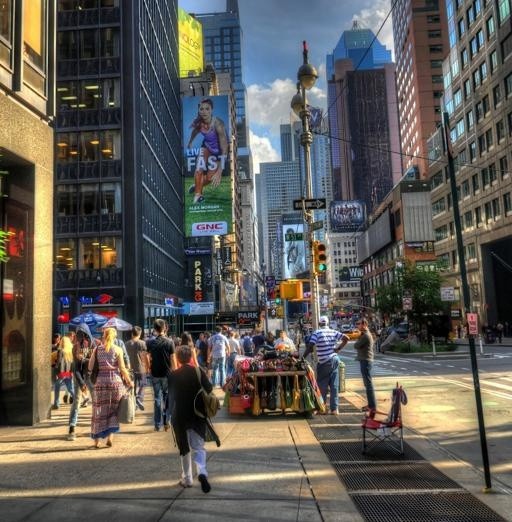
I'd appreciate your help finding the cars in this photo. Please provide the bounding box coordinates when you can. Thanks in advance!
[348,329,360,339]
[341,324,349,332]
[394,322,407,339]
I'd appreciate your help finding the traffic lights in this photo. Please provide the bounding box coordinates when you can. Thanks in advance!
[289,278,311,301]
[314,241,326,273]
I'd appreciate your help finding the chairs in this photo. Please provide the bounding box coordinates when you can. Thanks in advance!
[358,380,407,456]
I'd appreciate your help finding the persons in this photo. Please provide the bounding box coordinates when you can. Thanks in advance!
[162,344,222,494]
[451,320,506,345]
[303,316,350,414]
[188,101,229,210]
[51,311,312,450]
[354,316,375,421]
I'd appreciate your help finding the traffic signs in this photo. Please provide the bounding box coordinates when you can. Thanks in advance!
[293,198,326,210]
[310,220,324,231]
[285,233,302,241]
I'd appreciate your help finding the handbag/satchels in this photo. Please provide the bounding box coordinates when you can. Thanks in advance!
[90,360,98,384]
[118,386,136,423]
[223,350,326,413]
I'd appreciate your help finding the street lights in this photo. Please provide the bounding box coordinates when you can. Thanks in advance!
[289,39,321,332]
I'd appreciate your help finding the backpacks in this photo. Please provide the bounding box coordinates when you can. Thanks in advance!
[193,367,220,418]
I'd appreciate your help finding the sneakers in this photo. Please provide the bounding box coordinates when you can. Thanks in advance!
[198,474,210,493]
[137,397,145,410]
[188,183,205,205]
[52,395,88,441]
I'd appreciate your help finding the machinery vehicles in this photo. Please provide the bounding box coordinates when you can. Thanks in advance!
[320,316,329,329]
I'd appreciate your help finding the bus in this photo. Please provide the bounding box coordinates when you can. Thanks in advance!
[328,320,338,330]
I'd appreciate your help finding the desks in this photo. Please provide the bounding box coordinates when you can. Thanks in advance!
[224,356,327,417]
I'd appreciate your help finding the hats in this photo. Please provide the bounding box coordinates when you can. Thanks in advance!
[320,316,328,324]
